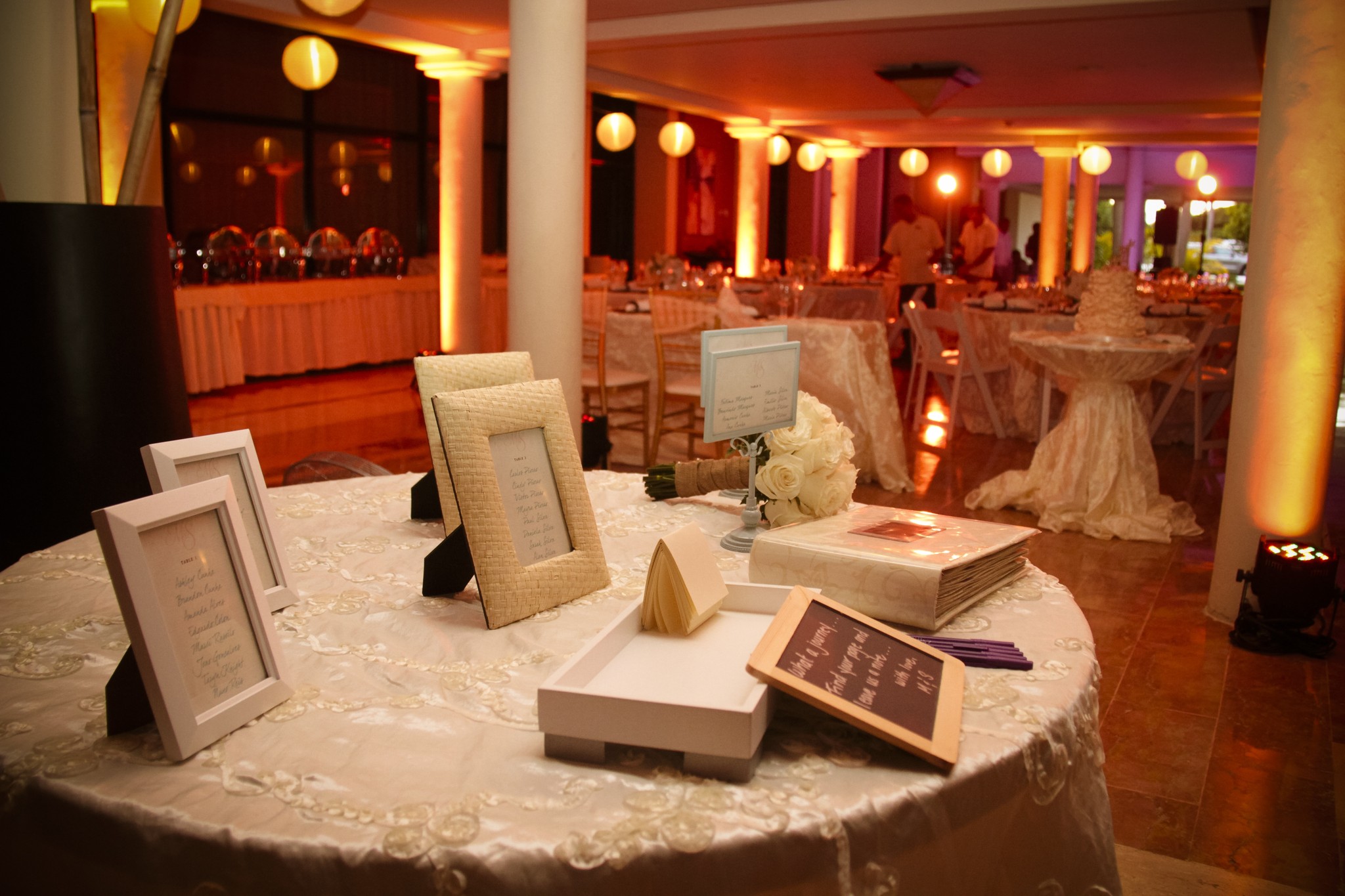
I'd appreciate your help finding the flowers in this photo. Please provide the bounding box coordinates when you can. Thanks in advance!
[644,386,860,528]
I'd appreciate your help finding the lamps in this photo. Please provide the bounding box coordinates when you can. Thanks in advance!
[1233,534,1342,662]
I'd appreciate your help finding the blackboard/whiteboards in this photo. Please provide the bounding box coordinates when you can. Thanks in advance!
[744,582,969,772]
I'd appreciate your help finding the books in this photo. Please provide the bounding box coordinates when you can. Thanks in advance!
[748,505,1043,630]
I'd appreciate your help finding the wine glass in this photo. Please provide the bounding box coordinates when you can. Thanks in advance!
[773,276,807,319]
[1040,286,1052,316]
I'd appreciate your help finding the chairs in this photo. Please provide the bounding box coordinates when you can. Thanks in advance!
[579,255,1245,497]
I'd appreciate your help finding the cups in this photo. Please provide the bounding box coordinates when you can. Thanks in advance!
[757,257,882,285]
[1007,277,1064,303]
[637,259,731,292]
[1137,272,1231,303]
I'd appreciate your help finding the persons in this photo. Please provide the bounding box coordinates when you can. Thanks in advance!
[1025,222,1039,264]
[995,217,1013,285]
[1012,250,1027,276]
[958,202,997,283]
[862,194,944,368]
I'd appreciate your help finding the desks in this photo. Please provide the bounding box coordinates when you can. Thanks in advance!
[962,303,1078,443]
[964,330,1202,547]
[1134,310,1204,359]
[732,282,906,358]
[176,271,461,396]
[588,295,714,370]
[760,313,914,494]
[1,466,1132,896]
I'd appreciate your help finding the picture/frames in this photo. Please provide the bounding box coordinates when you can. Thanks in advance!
[414,350,611,629]
[141,428,298,618]
[92,475,290,761]
